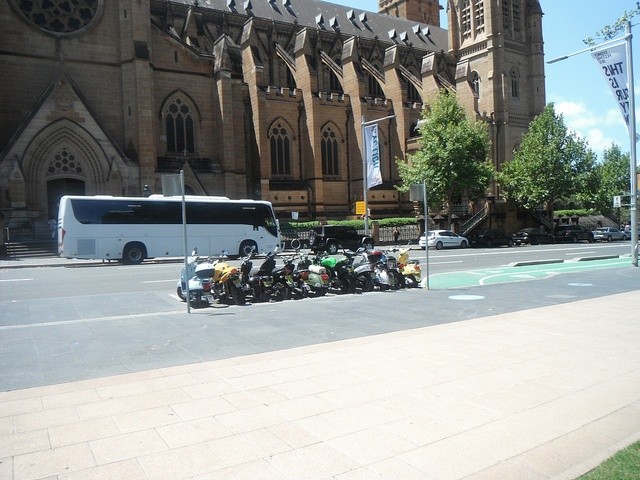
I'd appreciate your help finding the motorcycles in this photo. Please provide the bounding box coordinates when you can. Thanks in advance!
[176,247,216,307]
[377,249,406,290]
[208,249,245,305]
[314,250,356,293]
[251,251,295,302]
[293,252,330,298]
[396,248,422,288]
[356,240,396,291]
[239,245,274,302]
[339,247,382,292]
[280,254,311,300]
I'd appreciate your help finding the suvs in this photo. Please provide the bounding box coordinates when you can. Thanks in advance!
[308,225,375,255]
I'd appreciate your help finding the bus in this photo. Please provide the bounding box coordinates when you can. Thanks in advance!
[56,193,281,265]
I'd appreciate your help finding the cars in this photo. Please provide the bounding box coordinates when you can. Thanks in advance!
[418,229,469,250]
[592,226,626,241]
[511,228,555,245]
[469,229,513,248]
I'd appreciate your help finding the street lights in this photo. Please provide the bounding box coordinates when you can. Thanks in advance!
[361,114,397,236]
[545,20,638,268]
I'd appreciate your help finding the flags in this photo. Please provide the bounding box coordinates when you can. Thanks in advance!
[591,44,640,145]
[364,124,383,189]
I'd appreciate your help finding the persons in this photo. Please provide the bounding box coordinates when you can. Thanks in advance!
[625,224,631,238]
[393,226,400,244]
[48,216,57,240]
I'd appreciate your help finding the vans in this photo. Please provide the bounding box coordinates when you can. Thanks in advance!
[554,224,594,243]
